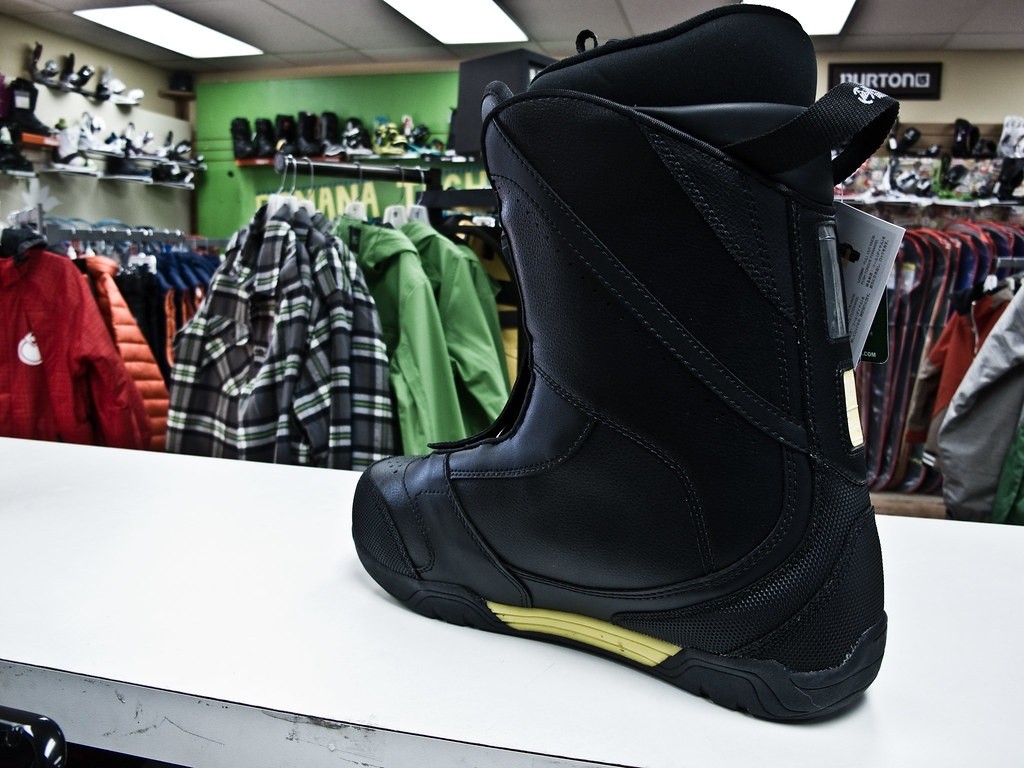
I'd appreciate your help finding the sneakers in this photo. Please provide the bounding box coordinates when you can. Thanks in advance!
[0,40,208,189]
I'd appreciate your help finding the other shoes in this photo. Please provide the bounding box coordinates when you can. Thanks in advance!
[231,109,480,163]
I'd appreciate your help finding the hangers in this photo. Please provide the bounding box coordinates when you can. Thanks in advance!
[0,156,432,261]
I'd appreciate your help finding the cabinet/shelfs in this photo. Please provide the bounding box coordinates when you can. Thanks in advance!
[0,133,474,180]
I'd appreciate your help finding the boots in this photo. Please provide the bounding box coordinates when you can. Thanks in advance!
[351,6,898,728]
